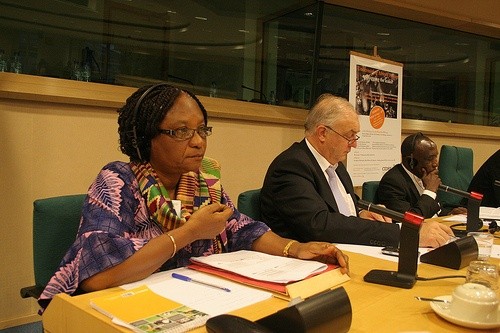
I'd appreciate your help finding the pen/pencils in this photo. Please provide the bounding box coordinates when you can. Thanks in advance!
[172,272,231,292]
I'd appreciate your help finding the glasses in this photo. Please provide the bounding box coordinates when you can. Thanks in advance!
[158,126,213,140]
[317,124,360,144]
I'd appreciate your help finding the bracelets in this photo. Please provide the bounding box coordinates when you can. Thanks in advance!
[283,240,299,257]
[165,232,176,258]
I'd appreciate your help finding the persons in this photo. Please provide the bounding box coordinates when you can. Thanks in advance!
[37,84,351,308]
[260,94,500,248]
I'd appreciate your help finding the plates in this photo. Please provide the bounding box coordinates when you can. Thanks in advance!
[430,295,500,329]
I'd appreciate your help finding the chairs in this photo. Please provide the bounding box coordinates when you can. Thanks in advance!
[238,187,263,222]
[362,181,380,203]
[435,144,475,208]
[19,193,88,300]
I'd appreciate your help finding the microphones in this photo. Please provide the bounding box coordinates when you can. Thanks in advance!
[241,85,269,104]
[494,180,500,188]
[167,75,194,86]
[86,46,101,73]
[439,185,483,203]
[356,199,423,231]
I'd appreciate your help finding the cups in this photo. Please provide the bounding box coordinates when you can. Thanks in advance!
[450,283,499,323]
[466,261,497,291]
[467,232,494,261]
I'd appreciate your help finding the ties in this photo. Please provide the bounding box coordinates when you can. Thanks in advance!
[325,167,351,216]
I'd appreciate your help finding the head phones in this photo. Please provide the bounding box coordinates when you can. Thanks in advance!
[406,132,424,171]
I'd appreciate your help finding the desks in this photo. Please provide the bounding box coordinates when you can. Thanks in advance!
[38,212,500,333]
